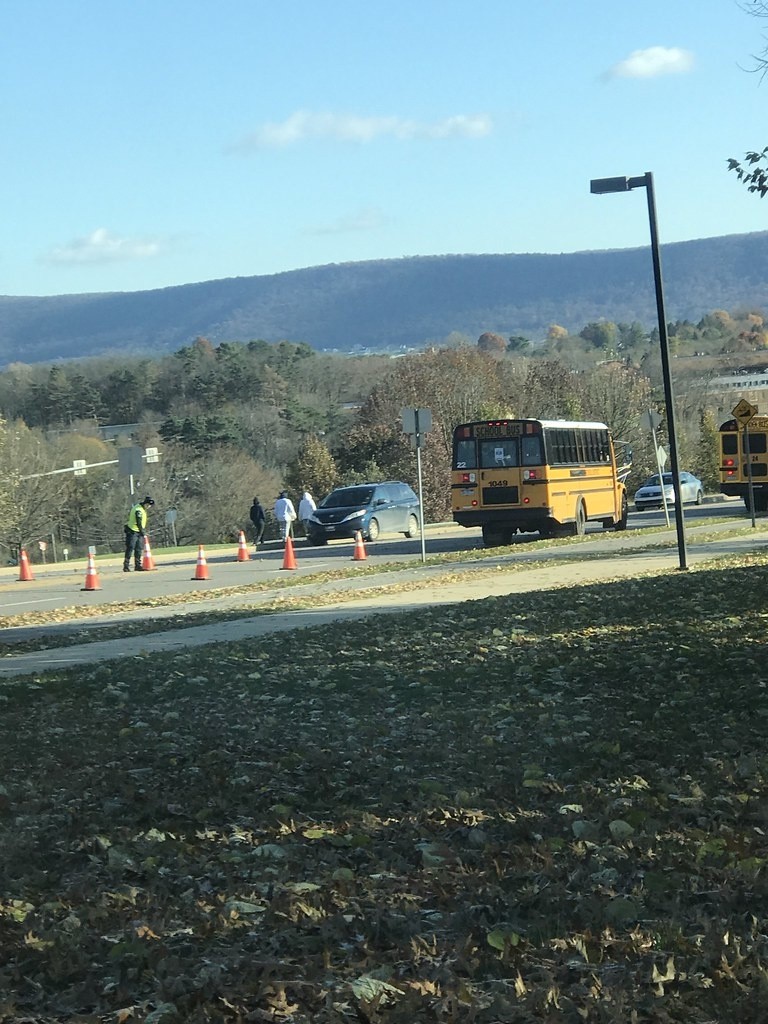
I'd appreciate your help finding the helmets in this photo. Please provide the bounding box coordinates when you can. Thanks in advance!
[144,496,154,503]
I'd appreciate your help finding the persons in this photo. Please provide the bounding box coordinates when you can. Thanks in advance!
[249,497,266,546]
[273,490,297,542]
[298,492,316,534]
[123,497,155,572]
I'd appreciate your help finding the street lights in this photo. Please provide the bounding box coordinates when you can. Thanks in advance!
[587,166,692,573]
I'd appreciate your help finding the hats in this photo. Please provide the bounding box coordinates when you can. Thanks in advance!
[280,492,288,498]
[253,498,258,503]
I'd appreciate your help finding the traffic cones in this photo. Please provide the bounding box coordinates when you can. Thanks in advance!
[16,545,35,583]
[139,532,159,572]
[351,528,368,563]
[190,543,210,582]
[78,545,103,592]
[281,534,298,572]
[234,529,252,564]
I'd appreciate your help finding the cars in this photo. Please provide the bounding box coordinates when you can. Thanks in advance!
[308,481,422,547]
[634,468,704,511]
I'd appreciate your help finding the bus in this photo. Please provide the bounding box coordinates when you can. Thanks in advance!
[450,418,632,547]
[717,415,768,513]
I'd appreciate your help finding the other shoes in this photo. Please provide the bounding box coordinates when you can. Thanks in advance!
[123,566,130,571]
[134,565,143,571]
